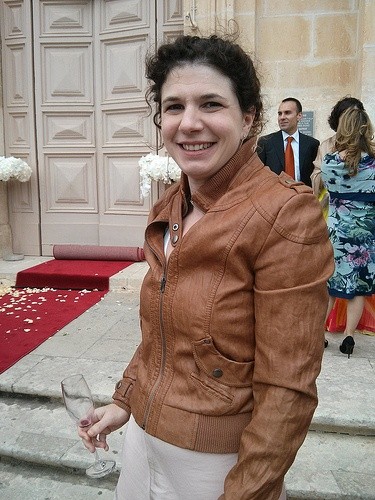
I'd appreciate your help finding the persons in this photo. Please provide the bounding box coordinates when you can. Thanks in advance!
[255,98,319,191]
[77,35,335,500]
[309,97,365,220]
[319,108,375,359]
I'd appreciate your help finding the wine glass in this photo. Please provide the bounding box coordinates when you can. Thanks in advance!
[60,374,116,478]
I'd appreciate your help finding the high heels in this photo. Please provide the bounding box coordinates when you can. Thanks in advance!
[339,336,355,359]
[323,340,328,348]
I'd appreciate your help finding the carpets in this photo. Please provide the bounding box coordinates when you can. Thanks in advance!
[0,244,145,373]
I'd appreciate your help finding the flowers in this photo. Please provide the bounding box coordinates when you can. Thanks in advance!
[0,156,33,183]
[138,151,182,199]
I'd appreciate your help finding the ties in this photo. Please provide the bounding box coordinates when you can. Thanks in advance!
[285,138,296,182]
